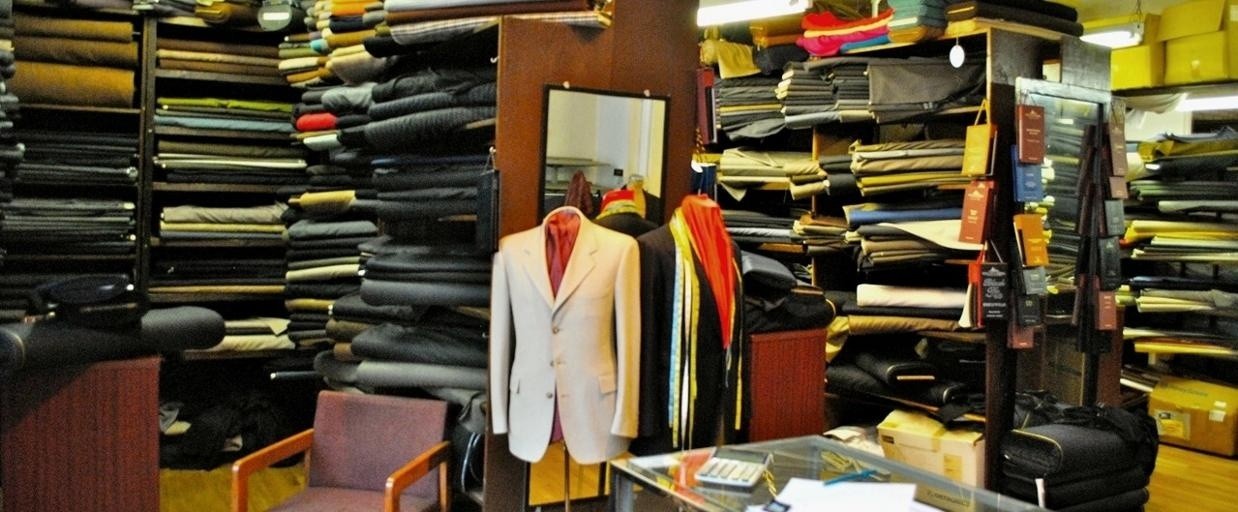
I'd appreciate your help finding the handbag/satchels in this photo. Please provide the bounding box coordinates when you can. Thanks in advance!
[958,101,1012,332]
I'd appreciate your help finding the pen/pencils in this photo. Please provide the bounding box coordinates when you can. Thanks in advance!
[823,470,877,485]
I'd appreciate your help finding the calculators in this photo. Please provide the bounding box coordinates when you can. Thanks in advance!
[694,446,773,488]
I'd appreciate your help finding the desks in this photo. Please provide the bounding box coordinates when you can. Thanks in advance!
[602,435,1045,512]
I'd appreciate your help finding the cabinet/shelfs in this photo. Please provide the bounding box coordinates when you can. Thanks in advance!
[435,3,709,512]
[0,0,316,512]
[723,14,1235,481]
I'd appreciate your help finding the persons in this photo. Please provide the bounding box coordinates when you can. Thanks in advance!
[596,189,655,237]
[619,175,661,220]
[488,202,641,468]
[635,195,749,460]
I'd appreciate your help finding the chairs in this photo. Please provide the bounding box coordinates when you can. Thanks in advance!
[222,385,454,512]
